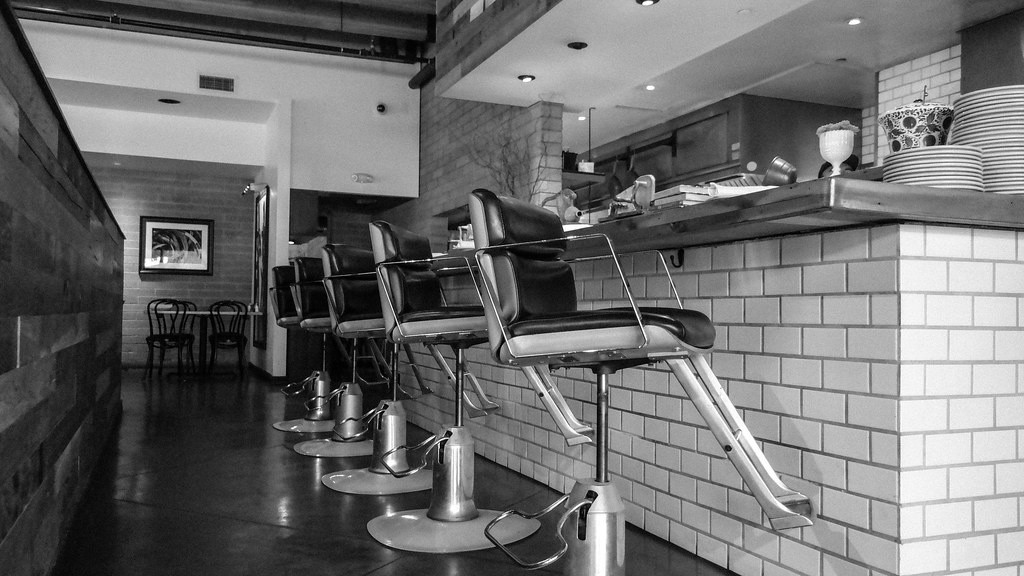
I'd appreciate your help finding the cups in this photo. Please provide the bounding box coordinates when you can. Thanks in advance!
[762,156,797,184]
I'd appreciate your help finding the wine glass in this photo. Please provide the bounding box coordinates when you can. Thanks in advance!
[819,130,854,176]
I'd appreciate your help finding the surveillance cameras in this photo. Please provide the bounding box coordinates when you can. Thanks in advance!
[375,102,388,116]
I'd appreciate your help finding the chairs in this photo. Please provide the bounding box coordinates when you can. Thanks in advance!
[321,243,498,495]
[290,257,433,456]
[142,299,248,381]
[368,219,595,553]
[270,266,388,432]
[469,189,814,575]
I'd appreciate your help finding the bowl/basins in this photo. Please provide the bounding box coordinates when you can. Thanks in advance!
[876,97,956,154]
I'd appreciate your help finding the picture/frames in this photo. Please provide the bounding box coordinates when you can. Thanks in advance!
[139,215,214,275]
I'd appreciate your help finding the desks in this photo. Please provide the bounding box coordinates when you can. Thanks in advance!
[144,310,263,377]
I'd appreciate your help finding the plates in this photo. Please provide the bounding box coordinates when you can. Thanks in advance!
[882,85,1024,194]
